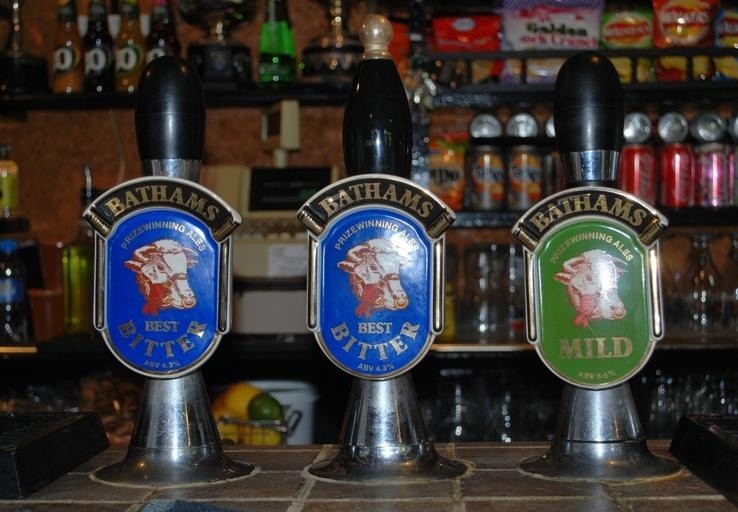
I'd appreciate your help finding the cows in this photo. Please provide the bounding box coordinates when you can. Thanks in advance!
[554,249,629,322]
[123,238,200,312]
[337,237,413,312]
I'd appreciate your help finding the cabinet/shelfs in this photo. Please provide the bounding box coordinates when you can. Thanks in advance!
[414,46,738,444]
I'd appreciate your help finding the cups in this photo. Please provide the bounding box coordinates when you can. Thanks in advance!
[648,371,737,434]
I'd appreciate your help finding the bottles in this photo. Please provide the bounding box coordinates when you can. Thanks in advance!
[50,0,178,93]
[258,0,295,87]
[0,143,95,347]
[455,242,525,343]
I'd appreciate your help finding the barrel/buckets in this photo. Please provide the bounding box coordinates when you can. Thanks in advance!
[251,378,317,444]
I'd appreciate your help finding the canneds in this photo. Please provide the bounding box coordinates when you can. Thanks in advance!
[465,110,737,210]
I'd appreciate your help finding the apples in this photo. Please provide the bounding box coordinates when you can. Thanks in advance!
[209,382,282,448]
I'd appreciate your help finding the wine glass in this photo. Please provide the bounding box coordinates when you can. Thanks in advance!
[664,230,738,333]
[435,362,524,443]
